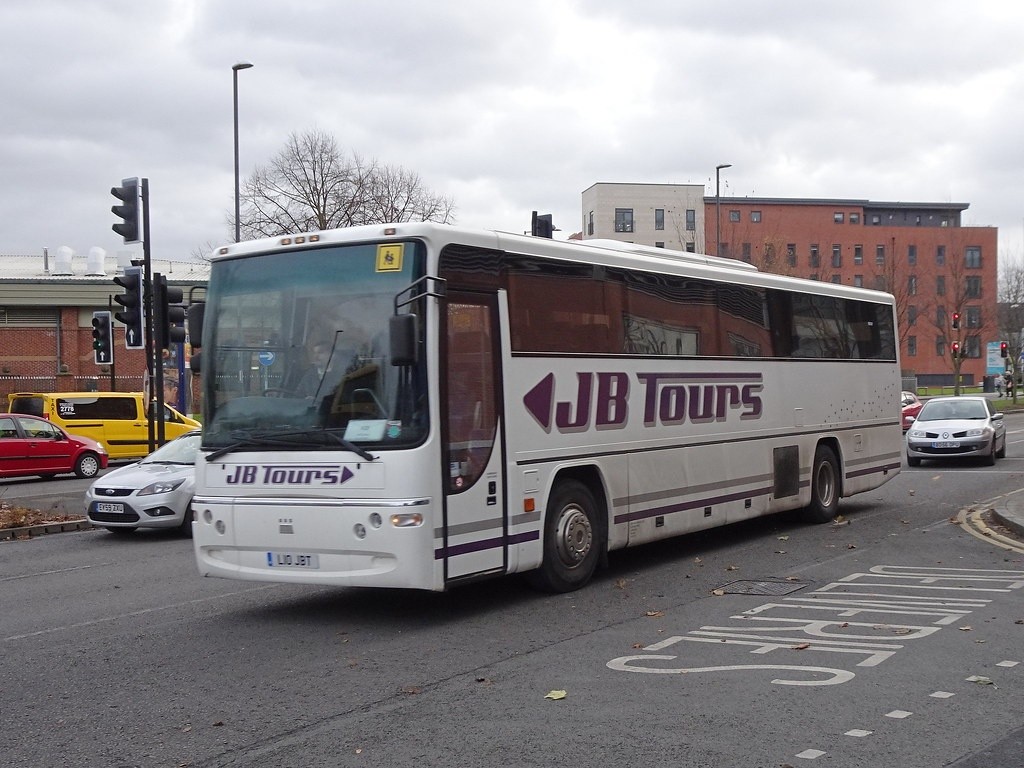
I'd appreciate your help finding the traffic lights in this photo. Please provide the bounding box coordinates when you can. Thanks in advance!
[1001,342,1007,358]
[110,177,142,243]
[953,342,959,357]
[92,312,112,364]
[953,312,959,327]
[113,267,146,349]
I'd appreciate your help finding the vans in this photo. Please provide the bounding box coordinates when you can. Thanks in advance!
[8,392,202,460]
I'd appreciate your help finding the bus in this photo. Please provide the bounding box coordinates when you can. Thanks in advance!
[192,222,909,596]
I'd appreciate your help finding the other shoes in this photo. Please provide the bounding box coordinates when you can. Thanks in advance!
[999,393,1003,397]
[1006,395,1010,397]
[1010,395,1013,397]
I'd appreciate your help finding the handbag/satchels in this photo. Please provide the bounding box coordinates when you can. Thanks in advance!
[1006,380,1012,389]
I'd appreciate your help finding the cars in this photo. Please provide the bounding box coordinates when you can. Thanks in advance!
[85,429,202,535]
[906,397,1005,467]
[0,413,109,478]
[900,392,923,430]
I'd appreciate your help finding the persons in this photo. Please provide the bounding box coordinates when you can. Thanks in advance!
[1004,371,1014,397]
[293,341,350,401]
[153,376,179,405]
[994,373,1003,397]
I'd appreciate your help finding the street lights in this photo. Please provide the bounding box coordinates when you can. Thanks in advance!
[716,163,731,256]
[229,60,252,243]
